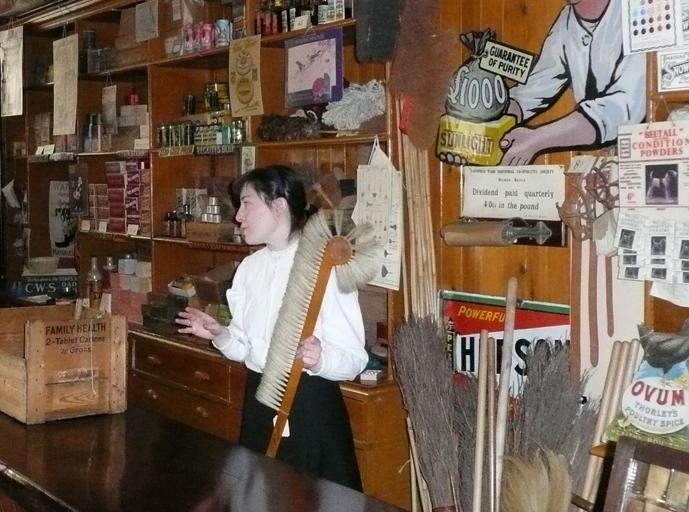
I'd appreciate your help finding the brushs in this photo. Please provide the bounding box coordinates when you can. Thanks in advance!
[254,209,383,460]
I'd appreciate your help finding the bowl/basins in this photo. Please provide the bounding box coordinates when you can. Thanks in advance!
[24,255,61,277]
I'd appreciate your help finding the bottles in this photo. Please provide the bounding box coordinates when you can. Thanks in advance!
[92,112,105,153]
[159,118,193,149]
[86,255,117,312]
[229,120,243,142]
[272,0,283,37]
[163,204,194,240]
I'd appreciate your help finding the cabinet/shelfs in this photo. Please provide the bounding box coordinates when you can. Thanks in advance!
[0,1,414,508]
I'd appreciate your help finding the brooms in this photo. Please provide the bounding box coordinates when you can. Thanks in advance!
[388,315,599,512]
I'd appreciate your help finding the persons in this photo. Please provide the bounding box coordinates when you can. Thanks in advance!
[171,163,372,494]
[436,1,649,172]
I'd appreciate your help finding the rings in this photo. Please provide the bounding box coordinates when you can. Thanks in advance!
[188,318,193,327]
